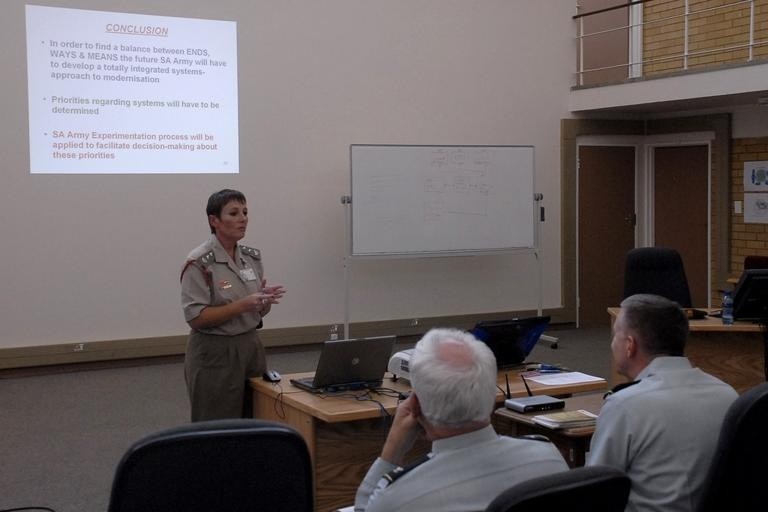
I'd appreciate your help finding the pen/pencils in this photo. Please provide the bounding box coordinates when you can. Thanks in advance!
[539,370,560,373]
[262,287,266,304]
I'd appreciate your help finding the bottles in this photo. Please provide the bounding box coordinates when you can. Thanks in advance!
[720,289,734,325]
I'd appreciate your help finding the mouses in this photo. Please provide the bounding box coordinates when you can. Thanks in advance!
[263,370,281,382]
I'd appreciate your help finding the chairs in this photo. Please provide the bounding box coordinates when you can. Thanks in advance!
[106,416,314,512]
[622,246,767,307]
[688,380,767,512]
[487,464,631,511]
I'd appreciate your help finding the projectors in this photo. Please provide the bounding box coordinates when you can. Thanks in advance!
[388,347,415,384]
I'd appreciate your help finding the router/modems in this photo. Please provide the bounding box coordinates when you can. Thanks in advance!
[504,373,566,413]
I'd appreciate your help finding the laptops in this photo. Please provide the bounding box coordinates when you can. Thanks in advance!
[473,315,551,369]
[709,268,768,319]
[290,335,397,394]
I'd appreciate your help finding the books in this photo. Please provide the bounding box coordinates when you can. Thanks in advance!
[532,409,599,430]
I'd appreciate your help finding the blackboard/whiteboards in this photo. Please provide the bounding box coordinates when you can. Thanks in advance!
[349,143,537,257]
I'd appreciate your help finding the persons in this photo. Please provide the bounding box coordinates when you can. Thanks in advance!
[178,187,285,424]
[583,293,740,512]
[353,324,571,511]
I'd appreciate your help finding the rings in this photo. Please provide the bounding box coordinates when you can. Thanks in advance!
[262,299,268,304]
